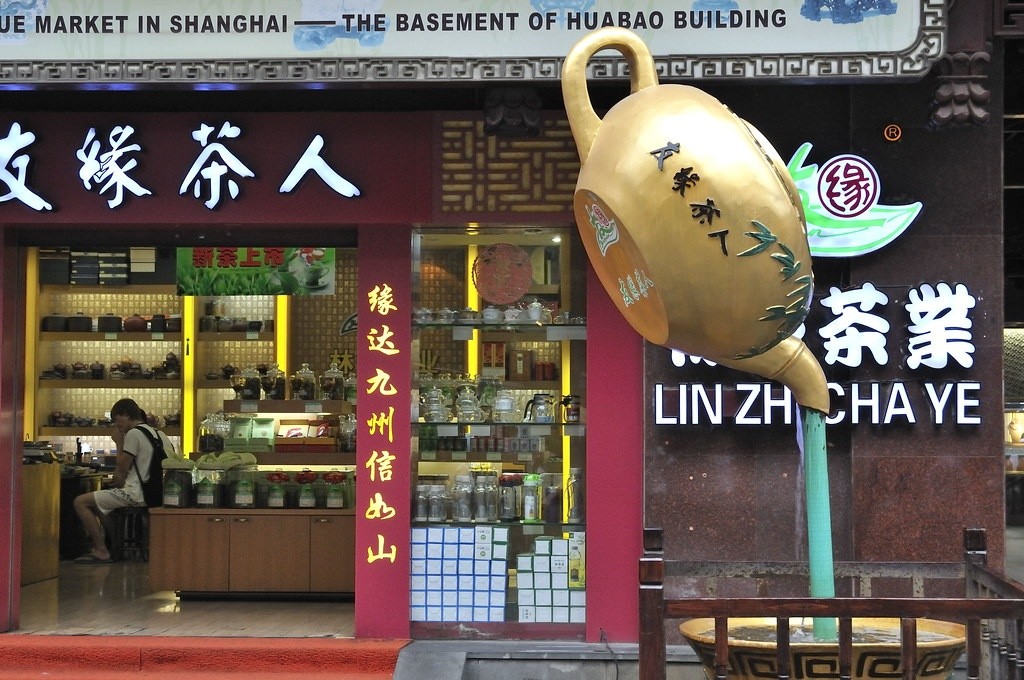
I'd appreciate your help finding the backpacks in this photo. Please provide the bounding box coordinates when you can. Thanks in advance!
[133,426,168,507]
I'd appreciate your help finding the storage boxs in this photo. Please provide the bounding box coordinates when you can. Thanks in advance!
[224,416,338,452]
[482,343,532,381]
[411,526,586,624]
[38,245,179,285]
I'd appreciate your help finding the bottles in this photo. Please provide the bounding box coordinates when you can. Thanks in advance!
[416,467,585,524]
[296,363,314,399]
[242,362,286,400]
[163,466,356,508]
[199,414,224,450]
[324,363,357,406]
[339,414,356,452]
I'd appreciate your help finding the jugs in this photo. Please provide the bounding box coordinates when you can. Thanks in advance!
[523,393,581,425]
[492,389,524,424]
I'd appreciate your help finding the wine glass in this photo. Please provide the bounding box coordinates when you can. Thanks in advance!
[260,376,271,401]
[229,375,243,400]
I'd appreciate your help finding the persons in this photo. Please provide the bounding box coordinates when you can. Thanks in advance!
[73,398,174,566]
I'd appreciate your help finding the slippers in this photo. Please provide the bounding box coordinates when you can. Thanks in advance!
[74,553,114,563]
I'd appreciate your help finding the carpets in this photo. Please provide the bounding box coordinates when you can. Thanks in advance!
[0,632,413,680]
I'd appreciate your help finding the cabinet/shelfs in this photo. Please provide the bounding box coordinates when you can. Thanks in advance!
[464,236,574,515]
[146,398,358,599]
[412,321,588,523]
[41,283,290,467]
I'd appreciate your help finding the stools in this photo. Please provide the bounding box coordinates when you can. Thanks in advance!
[109,507,146,560]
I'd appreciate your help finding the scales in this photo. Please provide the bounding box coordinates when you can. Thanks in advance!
[24,446,57,463]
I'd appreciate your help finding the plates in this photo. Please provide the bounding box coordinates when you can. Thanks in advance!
[458,319,480,324]
[414,319,433,324]
[436,319,455,324]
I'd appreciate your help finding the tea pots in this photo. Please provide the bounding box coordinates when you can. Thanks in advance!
[562,27,829,411]
[49,411,179,426]
[204,363,239,380]
[418,387,450,422]
[455,387,490,422]
[110,360,154,380]
[165,351,177,362]
[52,360,104,373]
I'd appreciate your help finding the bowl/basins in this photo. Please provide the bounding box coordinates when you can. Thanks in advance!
[678,618,966,679]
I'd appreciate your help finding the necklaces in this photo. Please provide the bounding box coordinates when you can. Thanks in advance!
[1009,424,1017,430]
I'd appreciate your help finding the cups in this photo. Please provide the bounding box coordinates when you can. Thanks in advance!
[415,298,547,320]
